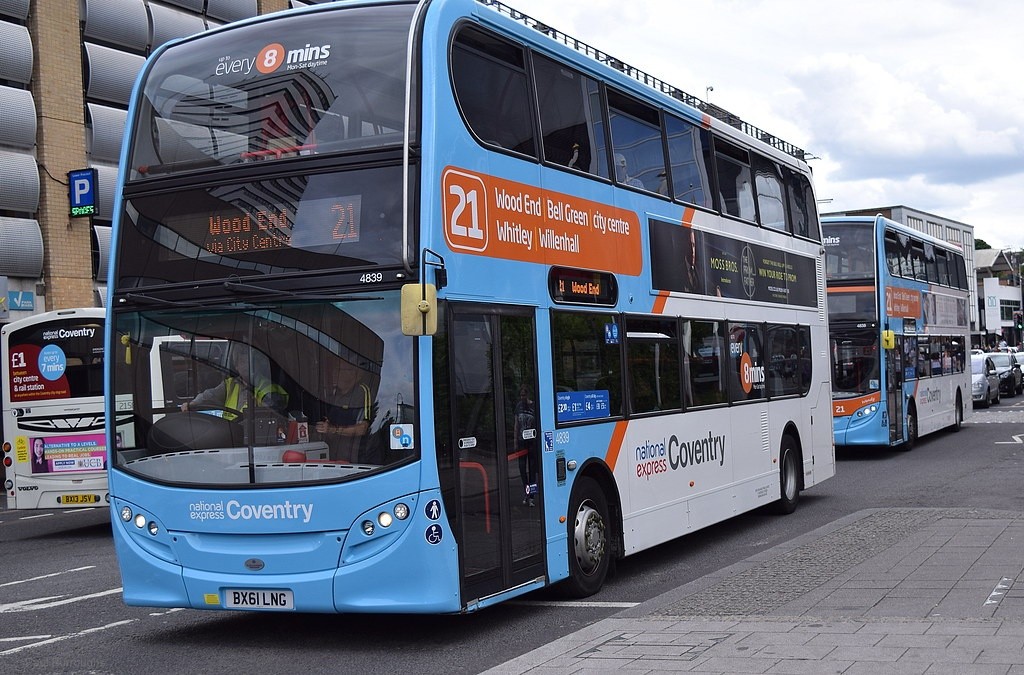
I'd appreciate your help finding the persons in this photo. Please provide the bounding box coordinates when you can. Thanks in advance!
[615,152,644,189]
[594,376,660,415]
[894,348,901,389]
[316,359,375,463]
[513,386,538,507]
[660,345,695,410]
[923,298,930,324]
[180,342,288,421]
[998,339,1007,347]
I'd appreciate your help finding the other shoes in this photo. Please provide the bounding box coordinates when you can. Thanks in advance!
[523,496,527,504]
[529,498,534,507]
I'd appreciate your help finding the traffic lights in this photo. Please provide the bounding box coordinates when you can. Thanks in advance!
[1013,313,1023,331]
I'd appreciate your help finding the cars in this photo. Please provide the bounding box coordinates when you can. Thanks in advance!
[971,346,1024,407]
[691,334,724,377]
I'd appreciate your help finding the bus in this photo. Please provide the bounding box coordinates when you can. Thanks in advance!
[101,0,837,617]
[762,212,973,451]
[0,306,228,512]
